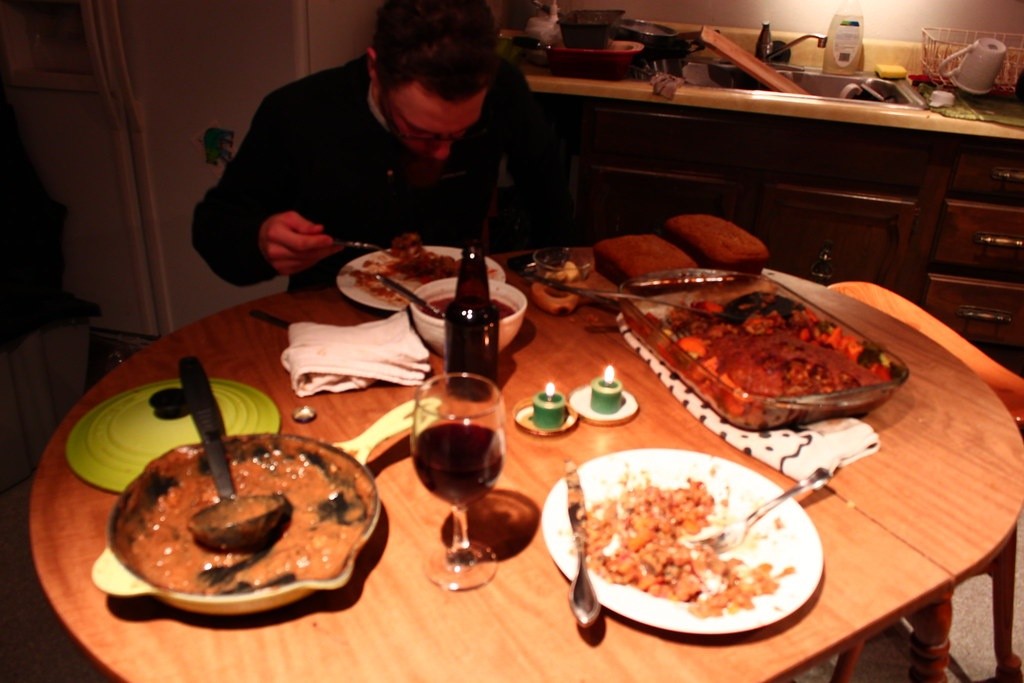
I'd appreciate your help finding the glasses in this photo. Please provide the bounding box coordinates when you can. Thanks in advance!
[378,77,489,143]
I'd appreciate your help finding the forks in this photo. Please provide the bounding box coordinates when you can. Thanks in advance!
[692,441,881,556]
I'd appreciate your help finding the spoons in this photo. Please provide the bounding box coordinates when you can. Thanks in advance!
[332,242,424,260]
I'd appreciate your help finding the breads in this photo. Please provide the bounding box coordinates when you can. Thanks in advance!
[592,233,702,297]
[662,214,769,284]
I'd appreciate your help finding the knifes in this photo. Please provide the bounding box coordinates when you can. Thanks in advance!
[566,461,600,626]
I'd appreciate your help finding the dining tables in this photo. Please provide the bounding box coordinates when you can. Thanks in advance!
[27,246,1024,683]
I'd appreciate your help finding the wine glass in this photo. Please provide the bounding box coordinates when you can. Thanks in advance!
[410,374,505,591]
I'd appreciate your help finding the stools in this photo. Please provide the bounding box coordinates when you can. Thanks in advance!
[829,282,1024,683]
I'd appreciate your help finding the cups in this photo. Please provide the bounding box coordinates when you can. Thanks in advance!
[939,37,1006,93]
[840,82,885,102]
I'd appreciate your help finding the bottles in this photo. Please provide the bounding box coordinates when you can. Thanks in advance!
[445,241,499,385]
[823,0,864,76]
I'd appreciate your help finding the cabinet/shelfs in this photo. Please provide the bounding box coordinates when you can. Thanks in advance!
[579,98,1024,377]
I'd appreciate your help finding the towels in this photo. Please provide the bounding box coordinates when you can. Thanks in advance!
[278,308,433,399]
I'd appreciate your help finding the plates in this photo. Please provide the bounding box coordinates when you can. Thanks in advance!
[619,268,909,431]
[542,448,824,635]
[336,245,506,310]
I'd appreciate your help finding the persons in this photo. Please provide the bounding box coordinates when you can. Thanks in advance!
[192,0,570,292]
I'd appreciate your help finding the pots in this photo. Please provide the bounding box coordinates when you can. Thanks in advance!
[511,37,645,79]
[92,399,415,614]
[633,38,705,75]
[613,18,679,60]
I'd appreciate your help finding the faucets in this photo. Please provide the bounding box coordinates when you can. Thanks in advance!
[755,19,829,59]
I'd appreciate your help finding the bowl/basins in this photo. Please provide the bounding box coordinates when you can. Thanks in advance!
[708,59,805,91]
[411,277,528,357]
[534,248,593,285]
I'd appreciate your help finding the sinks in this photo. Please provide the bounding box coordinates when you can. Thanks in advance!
[675,59,910,105]
